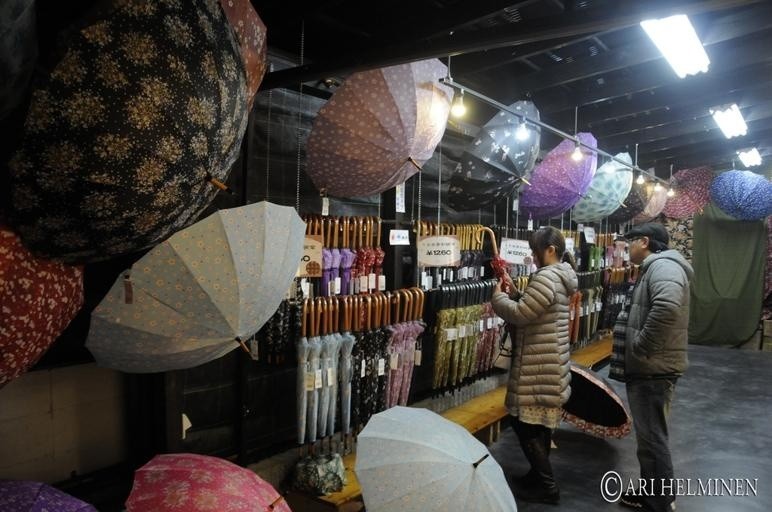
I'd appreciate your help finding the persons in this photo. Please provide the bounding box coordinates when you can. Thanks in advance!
[604,222,697,510]
[488,225,580,505]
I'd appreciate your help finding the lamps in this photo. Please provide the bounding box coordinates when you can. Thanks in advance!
[450,14,763,197]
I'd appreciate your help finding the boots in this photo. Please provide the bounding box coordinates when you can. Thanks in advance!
[507,434,558,505]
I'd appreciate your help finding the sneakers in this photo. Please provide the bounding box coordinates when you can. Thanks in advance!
[618,489,676,511]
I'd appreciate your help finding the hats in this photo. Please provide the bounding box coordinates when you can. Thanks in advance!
[623,221,668,244]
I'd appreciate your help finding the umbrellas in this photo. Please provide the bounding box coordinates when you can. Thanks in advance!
[1,477,100,511]
[0,226,86,393]
[446,99,541,212]
[518,131,598,221]
[218,1,270,117]
[2,1,249,264]
[353,405,519,512]
[125,452,294,511]
[85,200,309,374]
[606,166,656,224]
[662,164,714,219]
[630,184,667,223]
[305,58,455,200]
[709,159,772,222]
[570,151,634,224]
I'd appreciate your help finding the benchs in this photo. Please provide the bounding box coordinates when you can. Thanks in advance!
[292,331,616,506]
